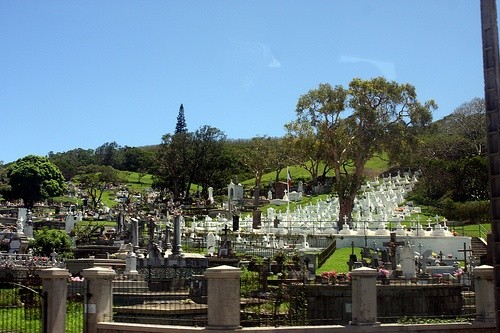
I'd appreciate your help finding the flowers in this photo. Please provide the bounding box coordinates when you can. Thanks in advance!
[321,267,465,286]
